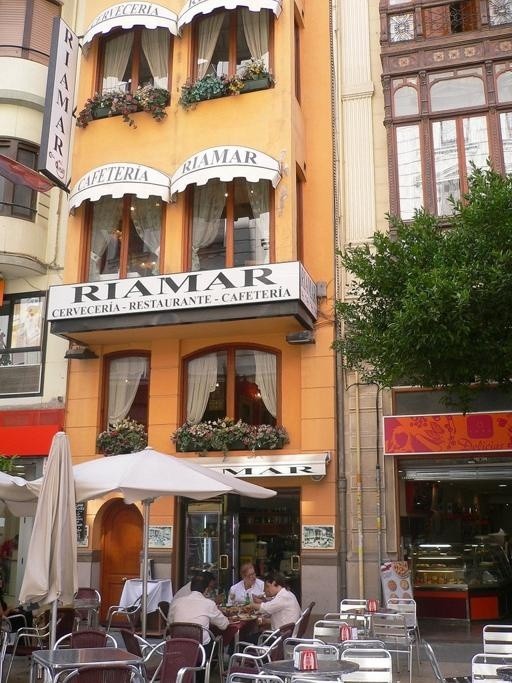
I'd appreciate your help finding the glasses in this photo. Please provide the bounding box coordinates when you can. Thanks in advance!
[243,572,257,578]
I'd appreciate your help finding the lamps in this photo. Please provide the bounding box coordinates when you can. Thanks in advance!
[284,330,318,345]
[63,347,100,359]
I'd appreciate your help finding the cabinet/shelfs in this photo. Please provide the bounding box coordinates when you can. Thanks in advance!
[408,540,511,623]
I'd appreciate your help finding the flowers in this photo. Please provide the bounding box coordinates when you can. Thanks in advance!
[74,56,275,132]
[168,415,293,463]
[92,413,149,457]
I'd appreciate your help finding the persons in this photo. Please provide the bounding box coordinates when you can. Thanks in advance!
[234,568,302,664]
[163,573,229,675]
[1,573,37,650]
[227,560,271,608]
[171,566,217,606]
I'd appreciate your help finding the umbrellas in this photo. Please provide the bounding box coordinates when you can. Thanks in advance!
[0,469,37,517]
[29,446,280,642]
[18,429,79,657]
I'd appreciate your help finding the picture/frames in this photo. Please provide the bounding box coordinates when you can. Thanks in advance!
[302,524,336,551]
[148,525,174,550]
[77,525,90,548]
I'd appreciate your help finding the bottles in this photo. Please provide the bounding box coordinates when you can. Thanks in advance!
[222,587,227,606]
[245,592,250,604]
